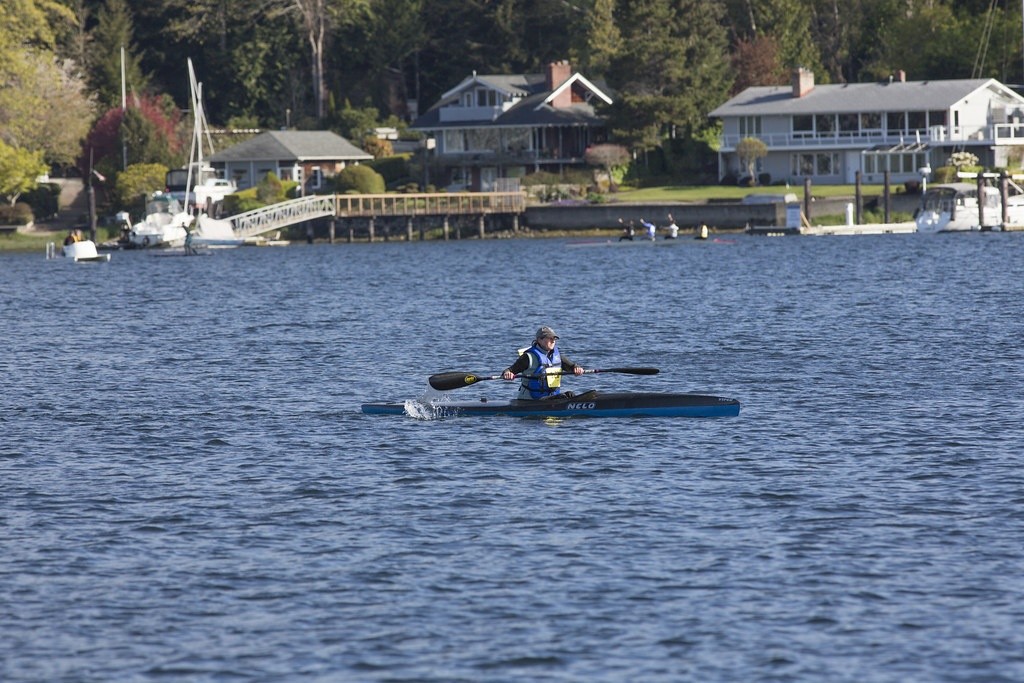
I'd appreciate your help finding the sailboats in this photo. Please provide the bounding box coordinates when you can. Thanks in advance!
[116,56,246,249]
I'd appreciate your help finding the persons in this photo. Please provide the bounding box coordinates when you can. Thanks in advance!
[178,222,192,254]
[695,221,707,240]
[64,228,84,245]
[119,225,132,242]
[618,218,635,241]
[501,327,584,399]
[639,219,655,240]
[660,220,680,238]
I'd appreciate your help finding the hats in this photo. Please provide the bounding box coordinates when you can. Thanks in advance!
[536,327,559,340]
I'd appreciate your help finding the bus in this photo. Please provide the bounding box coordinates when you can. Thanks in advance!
[919,182,1003,221]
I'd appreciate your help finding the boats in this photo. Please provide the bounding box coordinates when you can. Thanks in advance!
[60,240,111,262]
[361,390,740,417]
[152,251,215,256]
[564,239,730,247]
[240,231,291,247]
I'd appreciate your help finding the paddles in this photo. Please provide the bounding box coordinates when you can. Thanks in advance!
[428,366,661,392]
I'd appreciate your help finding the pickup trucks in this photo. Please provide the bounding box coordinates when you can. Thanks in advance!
[161,169,237,211]
